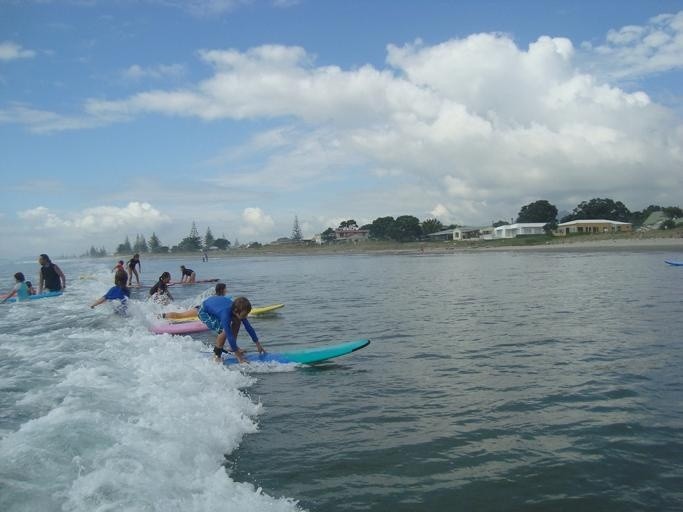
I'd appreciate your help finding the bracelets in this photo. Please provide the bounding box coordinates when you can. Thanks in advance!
[63,286,65,288]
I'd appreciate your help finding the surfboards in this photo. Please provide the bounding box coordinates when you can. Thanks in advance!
[201,338,369,365]
[127,282,175,288]
[169,279,220,284]
[149,320,209,334]
[166,304,284,321]
[0,290,63,304]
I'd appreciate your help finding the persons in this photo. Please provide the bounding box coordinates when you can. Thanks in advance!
[25,281,37,296]
[35,254,65,295]
[0,272,31,305]
[112,261,124,271]
[197,295,266,365]
[90,270,131,320]
[160,283,229,320]
[149,272,175,305]
[180,265,196,283]
[126,254,141,286]
